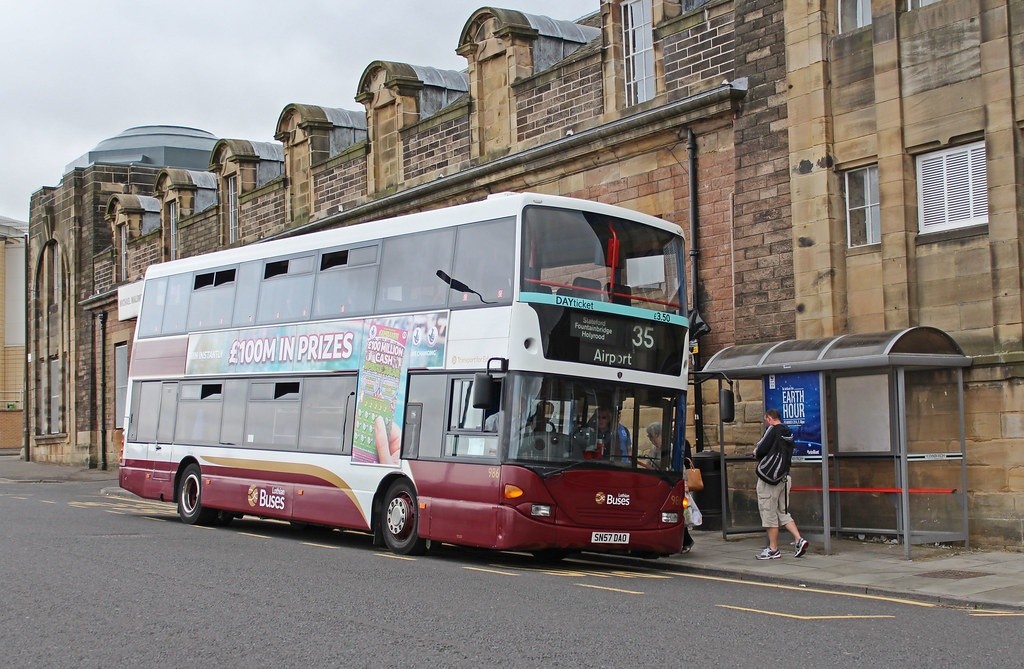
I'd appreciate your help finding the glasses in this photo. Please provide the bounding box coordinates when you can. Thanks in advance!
[595,415,608,420]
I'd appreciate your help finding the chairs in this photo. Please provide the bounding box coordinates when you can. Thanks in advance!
[534,277,631,306]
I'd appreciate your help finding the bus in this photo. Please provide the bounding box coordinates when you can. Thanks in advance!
[116,192,737,558]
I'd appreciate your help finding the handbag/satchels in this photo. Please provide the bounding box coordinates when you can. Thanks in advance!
[756,451,790,486]
[682,457,705,490]
[683,491,702,527]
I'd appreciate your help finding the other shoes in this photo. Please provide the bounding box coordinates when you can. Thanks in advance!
[679,541,696,554]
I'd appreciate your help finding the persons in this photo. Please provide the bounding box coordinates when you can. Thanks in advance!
[671,417,692,470]
[751,409,809,560]
[484,396,532,457]
[583,404,631,464]
[374,417,400,465]
[638,423,694,554]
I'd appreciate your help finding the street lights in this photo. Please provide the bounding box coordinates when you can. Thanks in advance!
[0,233,30,463]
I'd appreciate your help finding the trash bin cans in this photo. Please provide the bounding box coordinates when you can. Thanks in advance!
[692,451,732,531]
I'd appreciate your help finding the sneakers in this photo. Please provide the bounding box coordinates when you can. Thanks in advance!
[755,545,781,560]
[794,538,809,557]
